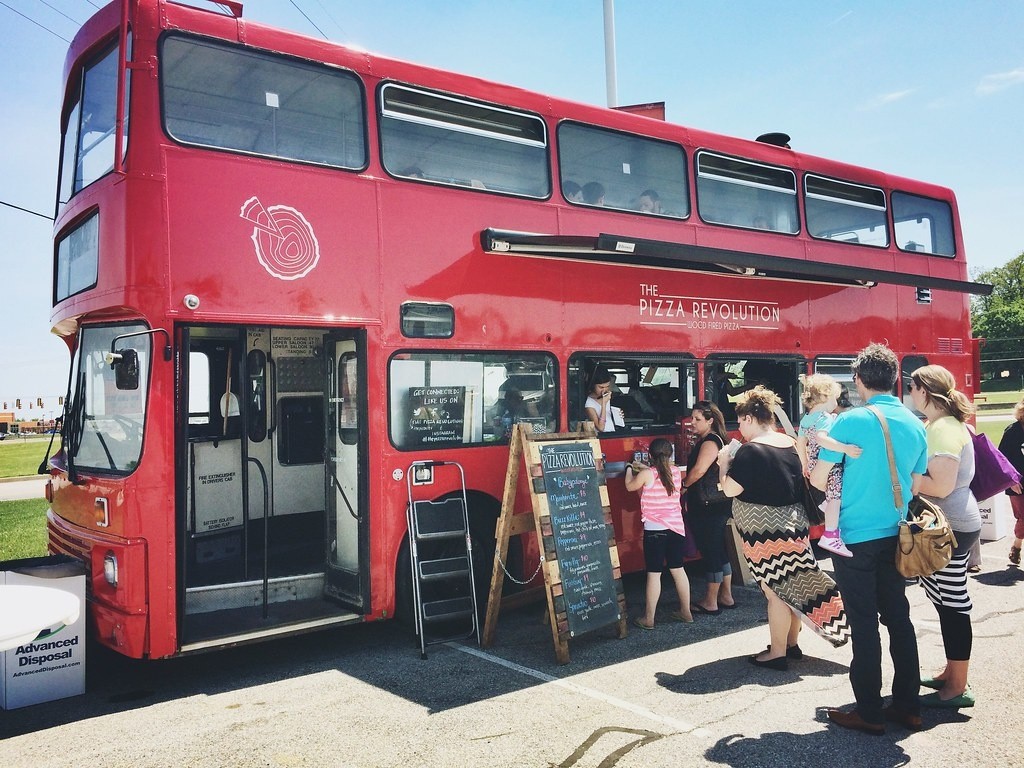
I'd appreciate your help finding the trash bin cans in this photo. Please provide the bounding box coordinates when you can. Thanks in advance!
[0,555,86,710]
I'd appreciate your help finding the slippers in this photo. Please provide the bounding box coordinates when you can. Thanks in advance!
[671,613,694,623]
[718,602,738,609]
[634,617,654,630]
[691,603,722,615]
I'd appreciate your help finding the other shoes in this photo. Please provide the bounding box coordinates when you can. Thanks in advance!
[748,650,787,671]
[967,564,981,572]
[1009,546,1021,564]
[921,676,971,690]
[920,688,975,708]
[767,644,802,659]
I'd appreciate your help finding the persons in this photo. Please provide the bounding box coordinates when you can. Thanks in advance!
[486,386,540,437]
[754,217,774,229]
[680,399,738,615]
[639,189,675,216]
[584,370,627,433]
[998,397,1024,565]
[624,438,695,629]
[795,337,987,735]
[562,181,605,207]
[717,385,852,671]
[401,167,424,179]
[719,365,762,407]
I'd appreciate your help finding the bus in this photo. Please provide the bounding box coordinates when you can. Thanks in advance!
[38,0,987,660]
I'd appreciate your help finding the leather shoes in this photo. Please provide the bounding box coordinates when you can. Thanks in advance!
[828,709,885,735]
[882,704,922,731]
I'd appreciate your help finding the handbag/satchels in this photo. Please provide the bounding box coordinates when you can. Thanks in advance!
[802,476,825,526]
[697,473,733,504]
[966,424,1023,503]
[895,495,958,578]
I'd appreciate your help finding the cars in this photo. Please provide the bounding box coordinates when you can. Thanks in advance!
[0,432,5,440]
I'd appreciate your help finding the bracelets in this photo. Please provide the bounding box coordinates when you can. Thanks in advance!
[681,478,689,489]
[598,416,607,422]
[625,465,633,472]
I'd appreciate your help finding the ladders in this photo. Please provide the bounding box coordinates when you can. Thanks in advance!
[405,462,481,657]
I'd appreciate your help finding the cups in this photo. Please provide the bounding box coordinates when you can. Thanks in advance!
[569,422,583,440]
[583,421,595,437]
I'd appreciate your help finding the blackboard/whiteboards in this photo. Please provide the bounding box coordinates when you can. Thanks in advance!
[525,432,625,640]
[407,386,464,446]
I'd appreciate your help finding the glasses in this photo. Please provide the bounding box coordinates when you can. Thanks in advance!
[907,383,923,393]
[853,374,856,382]
[700,401,714,415]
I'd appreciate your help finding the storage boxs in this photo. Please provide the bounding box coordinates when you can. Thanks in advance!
[0,569,87,711]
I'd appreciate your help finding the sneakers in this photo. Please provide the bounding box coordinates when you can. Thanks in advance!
[817,535,853,558]
